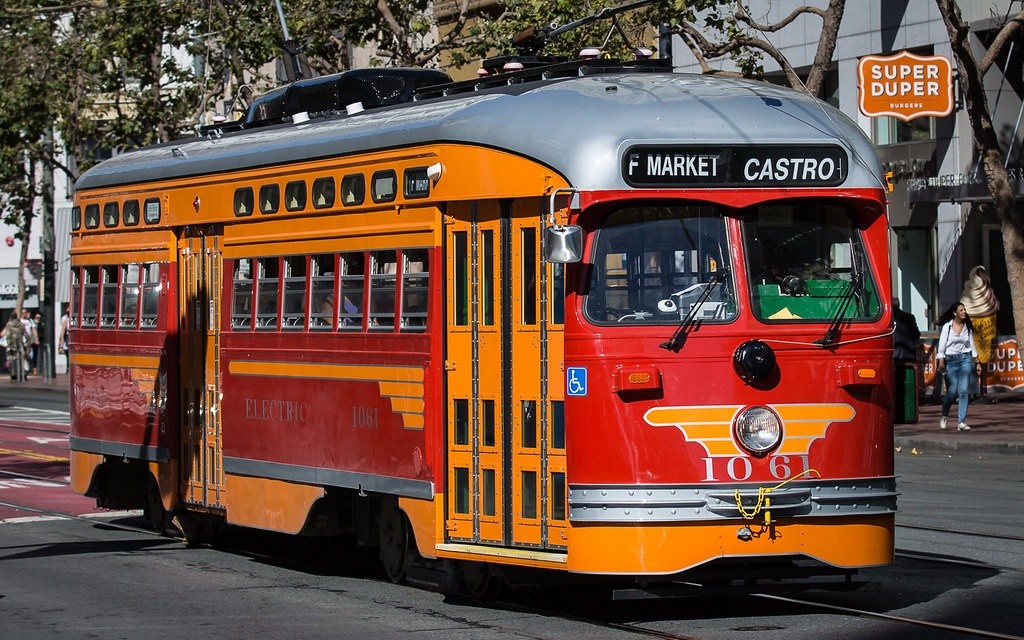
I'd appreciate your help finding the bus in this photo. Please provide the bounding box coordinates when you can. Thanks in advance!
[64,47,905,596]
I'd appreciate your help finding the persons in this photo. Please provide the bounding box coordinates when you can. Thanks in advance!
[889,297,922,423]
[0,309,41,380]
[937,302,982,431]
[59,304,69,373]
[6,312,30,382]
[320,281,362,327]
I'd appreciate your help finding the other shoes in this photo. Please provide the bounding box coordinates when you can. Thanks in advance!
[939,416,949,429]
[957,423,971,431]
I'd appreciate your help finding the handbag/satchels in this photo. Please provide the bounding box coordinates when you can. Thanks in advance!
[0,336,8,347]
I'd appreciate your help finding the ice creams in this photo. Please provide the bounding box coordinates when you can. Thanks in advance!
[960,266,1000,364]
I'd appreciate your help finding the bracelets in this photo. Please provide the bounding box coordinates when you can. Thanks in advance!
[976,361,981,365]
